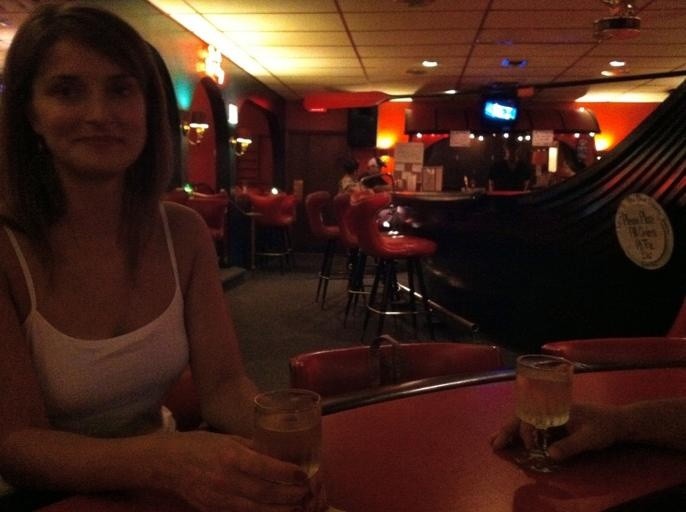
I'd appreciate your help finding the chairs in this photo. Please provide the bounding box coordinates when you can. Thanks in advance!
[169,181,303,282]
[542,336,685,371]
[305,189,357,307]
[350,190,436,342]
[287,344,508,397]
[336,186,423,327]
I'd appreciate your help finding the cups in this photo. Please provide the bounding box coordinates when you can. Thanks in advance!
[251,387,321,506]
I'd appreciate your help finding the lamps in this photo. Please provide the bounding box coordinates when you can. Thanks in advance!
[228,128,254,157]
[182,110,210,145]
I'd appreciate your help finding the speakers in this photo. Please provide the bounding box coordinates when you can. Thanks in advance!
[347,106,378,148]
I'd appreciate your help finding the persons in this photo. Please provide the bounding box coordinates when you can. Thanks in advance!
[489,143,531,191]
[0,5,328,511]
[338,157,395,272]
[492,397,685,462]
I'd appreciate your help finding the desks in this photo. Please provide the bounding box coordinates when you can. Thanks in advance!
[50,361,686,511]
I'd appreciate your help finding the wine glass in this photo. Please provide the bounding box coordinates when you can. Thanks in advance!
[513,353,570,473]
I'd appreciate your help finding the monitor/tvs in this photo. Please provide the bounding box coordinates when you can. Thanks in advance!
[479,95,521,124]
